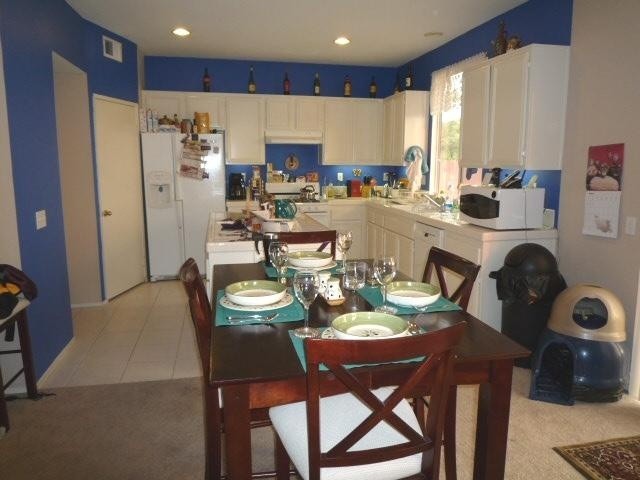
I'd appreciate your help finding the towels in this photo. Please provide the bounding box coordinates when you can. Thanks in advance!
[355,280,463,316]
[404,146,430,194]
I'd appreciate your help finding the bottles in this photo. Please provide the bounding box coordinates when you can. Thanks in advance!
[441,183,457,221]
[249,166,265,201]
[203,60,414,98]
[159,113,197,133]
[321,176,383,198]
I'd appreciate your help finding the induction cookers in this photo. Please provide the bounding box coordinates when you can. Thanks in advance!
[265,195,328,213]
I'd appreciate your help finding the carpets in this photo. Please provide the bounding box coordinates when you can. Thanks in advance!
[0,357,640,479]
[550,436,639,480]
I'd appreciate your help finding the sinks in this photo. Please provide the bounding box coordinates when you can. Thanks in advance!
[394,204,440,213]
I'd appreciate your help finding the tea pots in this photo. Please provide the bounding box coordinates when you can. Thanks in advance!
[300,184,318,201]
[272,199,298,221]
[386,170,400,188]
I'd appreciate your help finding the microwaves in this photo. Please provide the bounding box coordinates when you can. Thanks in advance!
[458,182,545,228]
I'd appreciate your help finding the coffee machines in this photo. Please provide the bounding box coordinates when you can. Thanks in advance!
[228,173,246,200]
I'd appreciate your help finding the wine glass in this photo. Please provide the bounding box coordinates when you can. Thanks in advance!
[336,230,354,274]
[268,242,289,284]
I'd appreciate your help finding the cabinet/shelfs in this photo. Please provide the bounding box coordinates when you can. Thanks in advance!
[330,201,365,260]
[460,43,570,171]
[139,91,384,166]
[383,90,430,166]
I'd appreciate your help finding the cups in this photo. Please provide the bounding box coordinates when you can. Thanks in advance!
[254,232,279,263]
[388,188,435,205]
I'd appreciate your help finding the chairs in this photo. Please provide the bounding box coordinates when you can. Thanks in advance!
[260,230,338,261]
[420,246,482,480]
[179,258,289,480]
[0,263,38,436]
[268,320,467,479]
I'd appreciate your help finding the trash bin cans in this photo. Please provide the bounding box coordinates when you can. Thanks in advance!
[489,243,569,368]
[527,284,629,406]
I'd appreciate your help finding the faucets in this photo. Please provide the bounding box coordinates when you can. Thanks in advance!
[420,193,446,211]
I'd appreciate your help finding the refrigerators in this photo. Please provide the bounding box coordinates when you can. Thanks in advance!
[138,133,227,283]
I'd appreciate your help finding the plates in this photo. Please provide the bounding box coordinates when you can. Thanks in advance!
[219,251,443,341]
